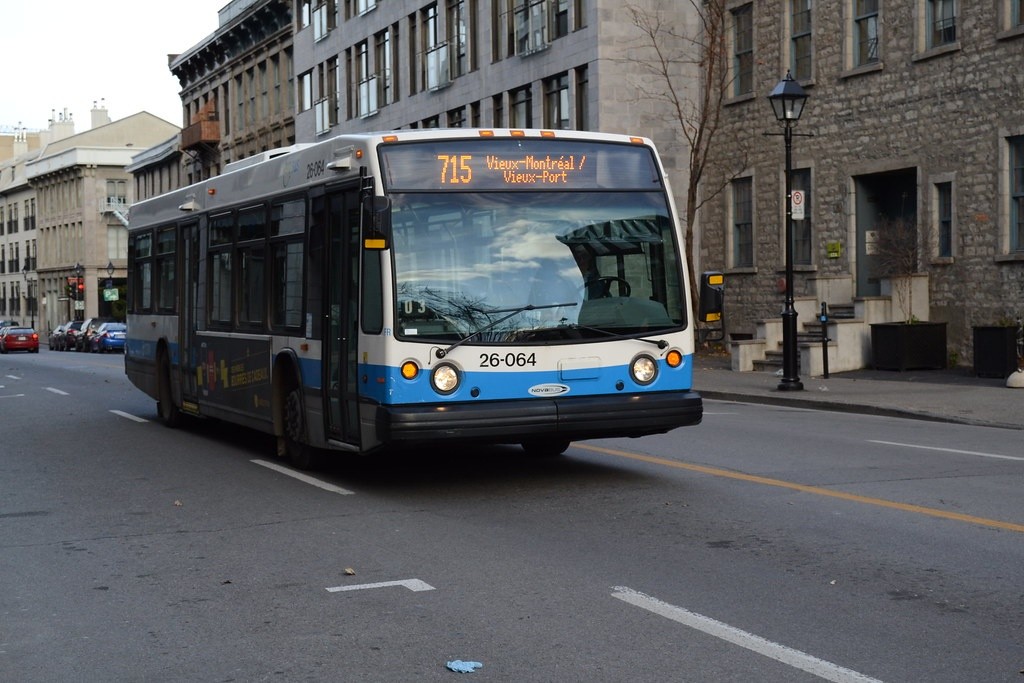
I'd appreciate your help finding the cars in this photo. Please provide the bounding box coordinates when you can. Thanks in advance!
[89,322,127,354]
[73,317,117,352]
[58,321,85,351]
[0,320,39,354]
[48,325,65,350]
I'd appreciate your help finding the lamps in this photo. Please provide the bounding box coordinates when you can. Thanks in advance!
[768,68,810,130]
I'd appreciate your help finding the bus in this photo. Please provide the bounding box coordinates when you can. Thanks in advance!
[124,127,724,476]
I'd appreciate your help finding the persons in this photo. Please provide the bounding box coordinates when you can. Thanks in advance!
[558,255,604,309]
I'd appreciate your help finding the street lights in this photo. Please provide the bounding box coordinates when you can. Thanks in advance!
[761,69,815,391]
[106,262,115,318]
[75,262,81,320]
[21,265,35,329]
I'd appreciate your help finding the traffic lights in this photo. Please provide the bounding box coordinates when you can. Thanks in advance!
[65,285,77,299]
[77,277,84,294]
